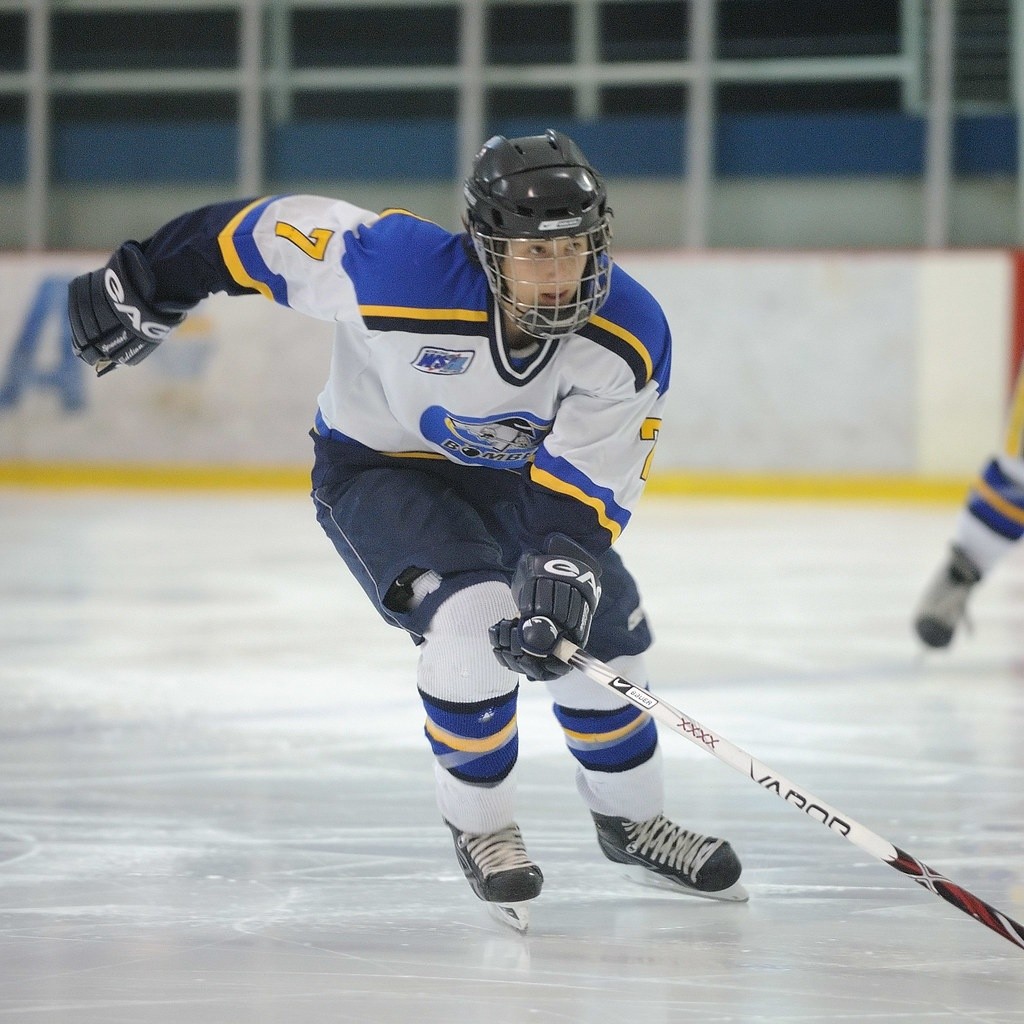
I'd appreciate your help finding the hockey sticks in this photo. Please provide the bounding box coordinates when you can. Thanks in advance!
[556,636,1024,953]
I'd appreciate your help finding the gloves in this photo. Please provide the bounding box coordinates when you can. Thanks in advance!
[67,239,199,377]
[488,533,602,682]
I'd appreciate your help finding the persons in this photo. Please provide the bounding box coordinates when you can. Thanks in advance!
[69,126,750,935]
[914,362,1024,670]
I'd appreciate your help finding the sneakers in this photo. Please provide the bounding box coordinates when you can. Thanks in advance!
[590,810,750,902]
[916,548,981,650]
[441,816,544,935]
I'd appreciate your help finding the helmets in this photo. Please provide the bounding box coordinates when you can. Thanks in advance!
[463,130,613,340]
